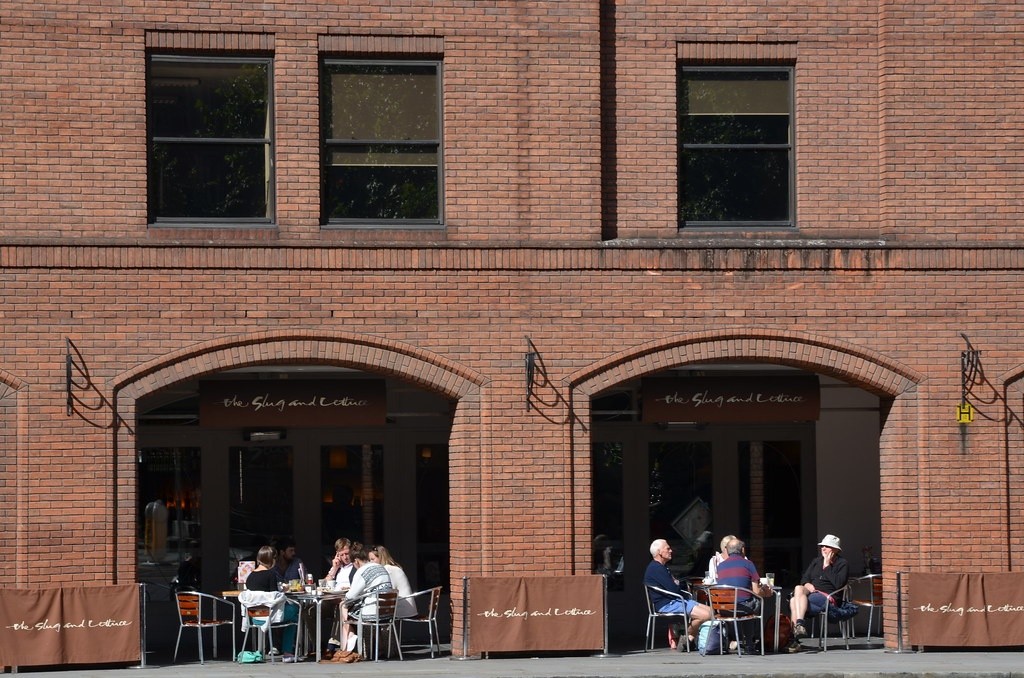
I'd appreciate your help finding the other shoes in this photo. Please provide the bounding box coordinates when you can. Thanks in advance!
[346,635,358,651]
[268,648,279,655]
[677,634,691,652]
[745,647,760,655]
[307,650,336,660]
[283,656,303,662]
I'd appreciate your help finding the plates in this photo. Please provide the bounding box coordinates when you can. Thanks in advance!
[284,591,305,594]
[324,589,348,594]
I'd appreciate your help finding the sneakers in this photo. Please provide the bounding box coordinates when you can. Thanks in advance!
[784,640,802,652]
[794,626,808,639]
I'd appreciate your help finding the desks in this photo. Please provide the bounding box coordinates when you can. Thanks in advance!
[213,590,347,664]
[680,581,782,657]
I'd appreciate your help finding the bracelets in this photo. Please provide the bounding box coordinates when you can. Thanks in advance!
[329,571,336,579]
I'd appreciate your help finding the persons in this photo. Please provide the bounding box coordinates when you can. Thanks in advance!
[644,536,773,652]
[246,538,418,663]
[788,534,849,652]
[688,531,717,575]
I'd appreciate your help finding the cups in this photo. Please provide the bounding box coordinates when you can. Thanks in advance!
[766,573,775,587]
[305,574,335,596]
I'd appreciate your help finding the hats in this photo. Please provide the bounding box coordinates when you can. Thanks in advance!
[817,535,841,551]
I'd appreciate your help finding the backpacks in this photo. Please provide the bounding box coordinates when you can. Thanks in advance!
[765,611,793,652]
[237,651,263,663]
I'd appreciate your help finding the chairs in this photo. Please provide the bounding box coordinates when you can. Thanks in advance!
[644,574,900,656]
[174,586,442,665]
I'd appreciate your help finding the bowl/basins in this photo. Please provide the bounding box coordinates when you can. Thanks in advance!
[705,579,714,584]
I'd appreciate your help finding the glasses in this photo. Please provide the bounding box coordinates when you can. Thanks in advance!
[820,545,831,548]
[371,546,378,551]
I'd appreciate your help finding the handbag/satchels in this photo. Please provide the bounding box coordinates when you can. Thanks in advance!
[331,650,359,662]
[668,624,681,649]
[696,621,727,656]
